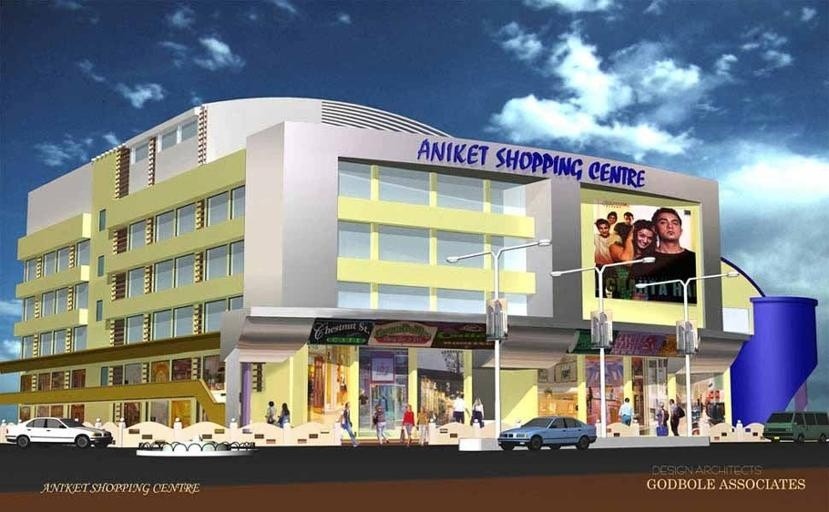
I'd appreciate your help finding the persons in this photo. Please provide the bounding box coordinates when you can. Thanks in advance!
[265,401,290,428]
[593,207,697,304]
[452,393,470,424]
[375,405,389,445]
[669,399,679,436]
[656,402,666,426]
[342,402,360,448]
[470,397,485,428]
[618,398,634,426]
[402,404,428,448]
